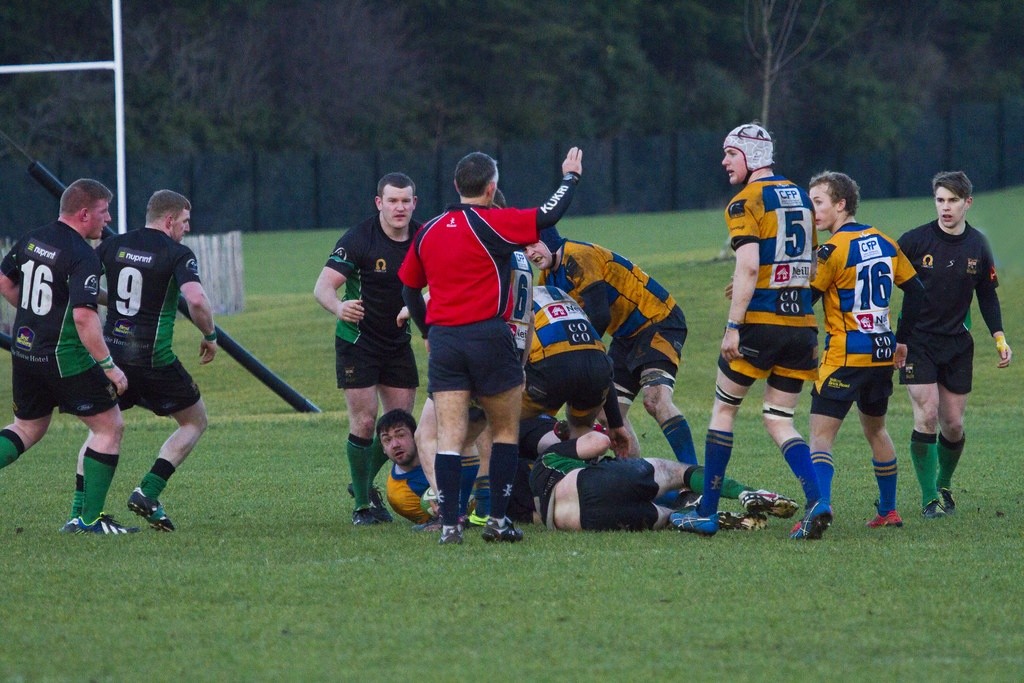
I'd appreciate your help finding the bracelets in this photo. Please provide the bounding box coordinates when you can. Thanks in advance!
[204,330,217,341]
[97,355,114,368]
[562,174,579,184]
[727,318,742,331]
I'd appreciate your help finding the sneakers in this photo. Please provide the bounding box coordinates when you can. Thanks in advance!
[75,512,142,535]
[788,502,834,540]
[670,508,719,537]
[553,419,603,441]
[481,516,524,543]
[58,517,78,535]
[678,487,692,500]
[739,489,799,519]
[684,493,703,509]
[127,487,174,533]
[716,510,769,533]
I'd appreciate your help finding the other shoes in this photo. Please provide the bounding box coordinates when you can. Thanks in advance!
[352,508,376,527]
[347,482,355,497]
[458,514,470,529]
[410,518,443,533]
[937,487,955,511]
[368,484,394,522]
[468,509,490,527]
[865,498,903,527]
[438,527,463,544]
[921,499,948,518]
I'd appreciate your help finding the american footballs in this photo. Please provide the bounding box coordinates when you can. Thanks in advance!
[420,485,477,519]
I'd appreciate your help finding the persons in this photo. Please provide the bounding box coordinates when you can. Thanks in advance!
[723,172,927,536]
[894,172,1012,520]
[398,146,583,544]
[59,190,217,532]
[670,123,834,536]
[379,189,798,532]
[0,179,140,536]
[311,173,433,522]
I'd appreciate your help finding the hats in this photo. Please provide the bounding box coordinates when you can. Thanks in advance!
[723,123,775,172]
[540,226,566,255]
